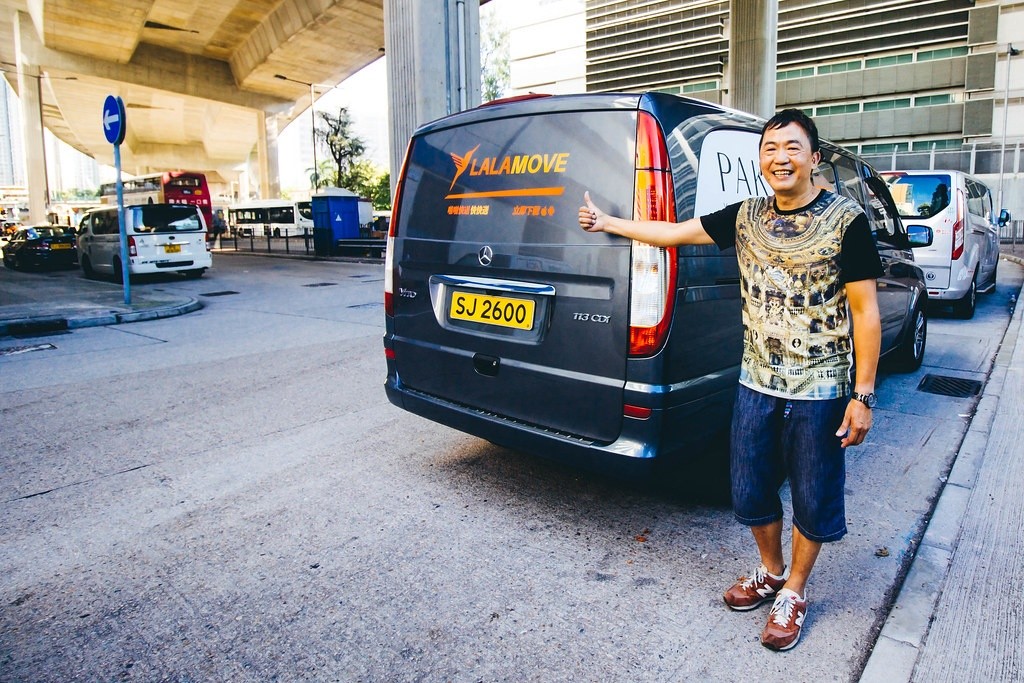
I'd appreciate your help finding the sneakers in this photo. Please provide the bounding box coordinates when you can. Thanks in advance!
[761,588,808,651]
[723,562,790,611]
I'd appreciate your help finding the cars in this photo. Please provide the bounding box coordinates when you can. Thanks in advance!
[2,227,79,272]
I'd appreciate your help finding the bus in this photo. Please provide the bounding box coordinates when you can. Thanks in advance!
[99,171,212,250]
[226,195,315,239]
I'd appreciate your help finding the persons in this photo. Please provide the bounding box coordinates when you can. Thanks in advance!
[579,109,881,649]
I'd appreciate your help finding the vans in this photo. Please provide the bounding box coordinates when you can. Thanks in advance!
[878,170,1011,319]
[2,221,25,237]
[69,200,214,284]
[383,95,927,482]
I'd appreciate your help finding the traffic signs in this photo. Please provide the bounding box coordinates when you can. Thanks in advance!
[102,95,125,144]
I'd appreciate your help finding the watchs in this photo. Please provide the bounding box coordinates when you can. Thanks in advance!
[851,392,878,409]
